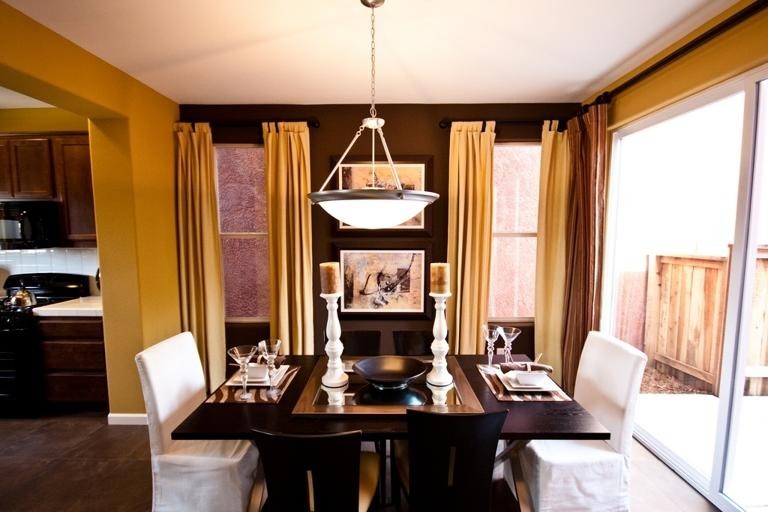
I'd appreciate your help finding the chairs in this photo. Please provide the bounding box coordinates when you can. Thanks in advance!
[246,424,363,512]
[321,317,438,455]
[402,408,507,511]
[133,330,263,512]
[519,327,647,512]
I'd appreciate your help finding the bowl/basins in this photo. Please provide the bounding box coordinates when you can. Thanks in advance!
[353,385,428,405]
[350,354,427,390]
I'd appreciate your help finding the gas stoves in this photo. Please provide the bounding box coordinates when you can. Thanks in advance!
[0,298,67,328]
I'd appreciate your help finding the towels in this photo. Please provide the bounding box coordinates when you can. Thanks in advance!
[500,361,553,374]
[230,354,286,368]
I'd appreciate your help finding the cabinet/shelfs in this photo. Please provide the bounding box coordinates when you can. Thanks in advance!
[1,134,53,200]
[56,134,100,242]
[31,295,110,418]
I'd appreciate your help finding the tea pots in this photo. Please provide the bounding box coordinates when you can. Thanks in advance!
[10,281,36,308]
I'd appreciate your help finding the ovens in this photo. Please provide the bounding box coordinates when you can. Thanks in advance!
[0,329,45,416]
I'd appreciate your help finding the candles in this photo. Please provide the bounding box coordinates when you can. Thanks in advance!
[429,262,452,294]
[319,262,341,294]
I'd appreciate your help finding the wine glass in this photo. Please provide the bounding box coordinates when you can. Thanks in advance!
[482,324,500,369]
[257,338,282,398]
[497,326,522,362]
[226,346,257,401]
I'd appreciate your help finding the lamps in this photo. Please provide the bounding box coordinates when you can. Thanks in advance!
[305,0,440,231]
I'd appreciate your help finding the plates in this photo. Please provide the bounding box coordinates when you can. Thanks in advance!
[226,362,291,387]
[497,370,560,394]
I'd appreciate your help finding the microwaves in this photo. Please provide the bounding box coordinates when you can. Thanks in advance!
[0,200,69,250]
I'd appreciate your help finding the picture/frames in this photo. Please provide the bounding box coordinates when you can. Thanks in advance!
[337,163,427,231]
[338,250,426,314]
